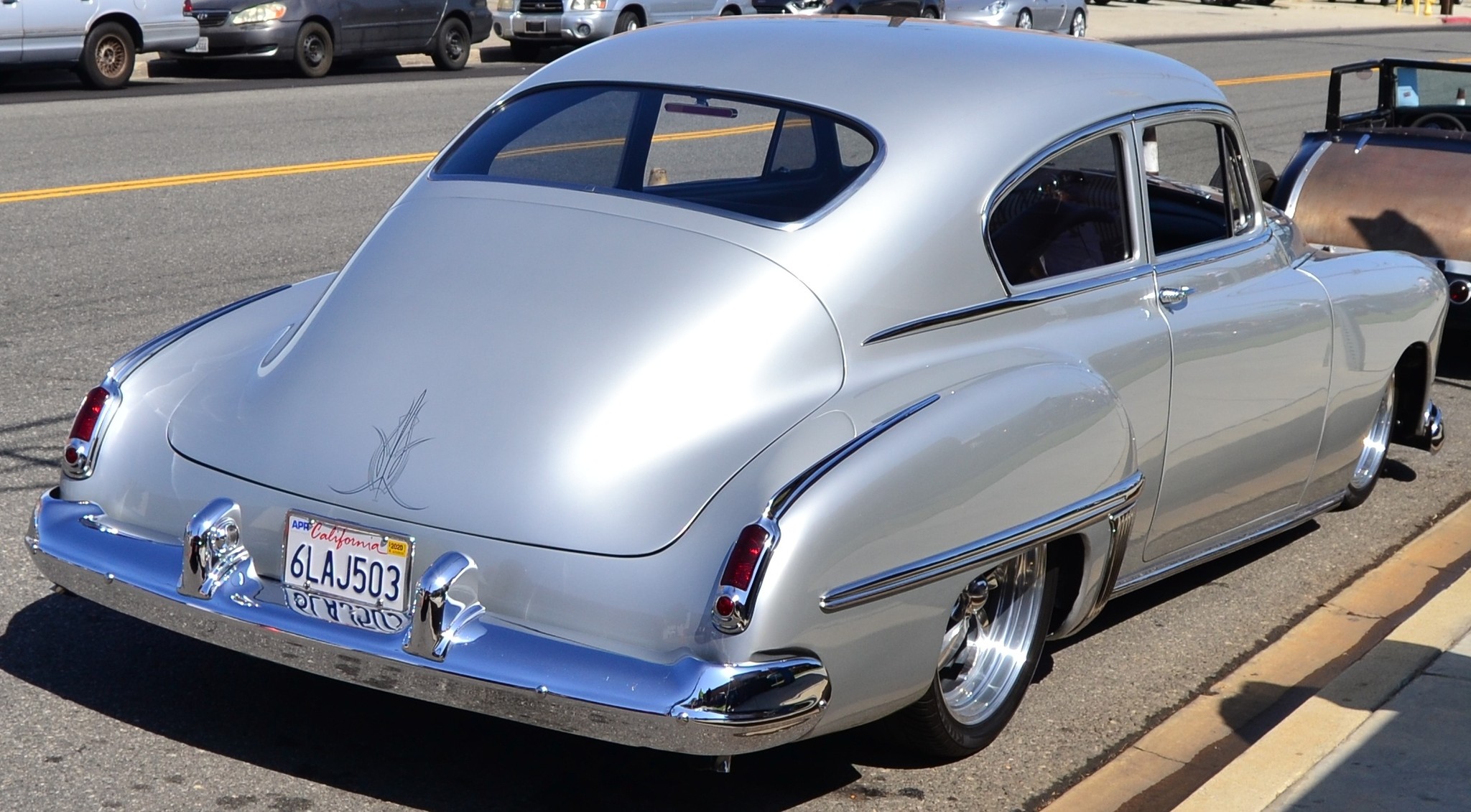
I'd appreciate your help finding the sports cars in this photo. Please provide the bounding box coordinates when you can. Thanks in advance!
[1208,56,1471,335]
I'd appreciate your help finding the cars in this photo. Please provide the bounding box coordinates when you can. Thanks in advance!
[158,1,496,79]
[1,1,199,89]
[25,7,1454,756]
[491,0,762,48]
[781,1,1089,39]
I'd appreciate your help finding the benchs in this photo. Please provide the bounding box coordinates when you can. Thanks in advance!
[1013,168,1230,252]
